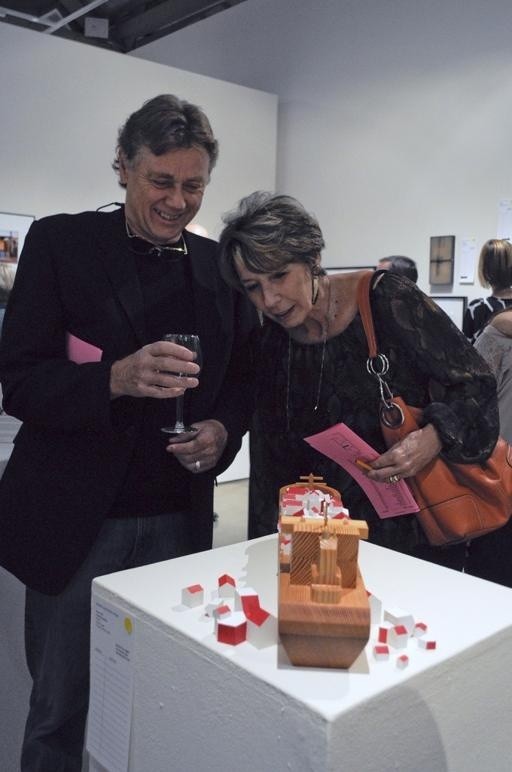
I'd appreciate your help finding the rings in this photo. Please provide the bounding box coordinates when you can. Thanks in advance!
[389,475,399,484]
[196,461,201,471]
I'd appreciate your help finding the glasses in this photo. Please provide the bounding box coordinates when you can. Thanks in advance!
[122,213,189,263]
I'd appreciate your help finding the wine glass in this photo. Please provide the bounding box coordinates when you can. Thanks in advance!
[159,334,206,432]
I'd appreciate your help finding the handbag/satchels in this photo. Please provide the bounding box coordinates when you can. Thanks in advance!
[352,268,512,547]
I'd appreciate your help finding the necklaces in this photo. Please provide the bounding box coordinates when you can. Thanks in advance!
[287,325,327,417]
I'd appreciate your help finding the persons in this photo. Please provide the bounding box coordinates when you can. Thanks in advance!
[460,239,512,346]
[471,305,512,447]
[0,93,264,771]
[220,190,502,578]
[374,256,418,283]
